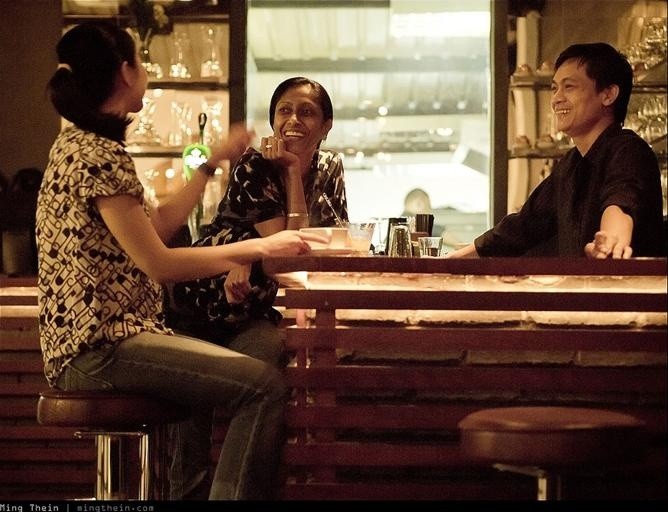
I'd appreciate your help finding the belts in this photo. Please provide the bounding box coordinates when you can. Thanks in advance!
[618,19,668,143]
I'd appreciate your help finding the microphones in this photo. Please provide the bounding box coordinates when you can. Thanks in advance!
[36,387,207,504]
[458,406,652,504]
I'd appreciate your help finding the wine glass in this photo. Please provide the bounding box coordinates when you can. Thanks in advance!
[265,145,272,149]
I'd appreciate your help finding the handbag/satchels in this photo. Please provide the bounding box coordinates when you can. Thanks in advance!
[198,163,213,175]
[286,213,308,217]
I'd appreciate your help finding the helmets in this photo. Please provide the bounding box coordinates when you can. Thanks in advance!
[512,72,532,78]
[536,72,554,77]
[512,144,531,150]
[535,143,555,149]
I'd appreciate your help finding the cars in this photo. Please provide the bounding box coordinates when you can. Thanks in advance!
[418,236,443,258]
[346,223,376,252]
[128,26,223,208]
[297,227,347,250]
[385,218,412,257]
[412,241,420,258]
[414,213,434,236]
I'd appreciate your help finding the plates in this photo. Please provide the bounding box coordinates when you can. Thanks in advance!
[506,1,666,261]
[84,10,242,256]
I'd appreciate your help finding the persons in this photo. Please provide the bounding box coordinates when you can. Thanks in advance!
[34,23,328,501]
[172,77,348,331]
[448,42,663,260]
